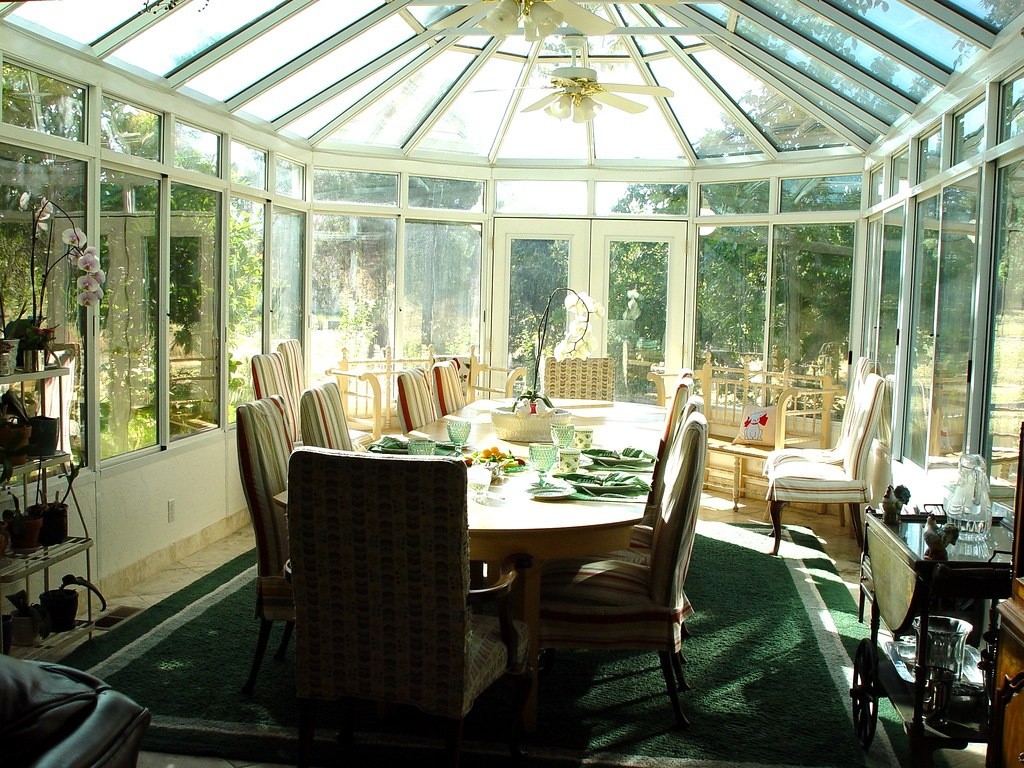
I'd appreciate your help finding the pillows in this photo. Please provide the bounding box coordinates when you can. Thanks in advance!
[731,403,777,446]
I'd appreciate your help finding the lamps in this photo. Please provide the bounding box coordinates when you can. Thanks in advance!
[478,0,564,42]
[545,67,604,121]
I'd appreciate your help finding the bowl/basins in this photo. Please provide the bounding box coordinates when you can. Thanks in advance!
[471,462,499,481]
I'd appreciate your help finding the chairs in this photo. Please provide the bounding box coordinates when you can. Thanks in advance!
[765,373,885,556]
[761,356,873,538]
[237,338,709,768]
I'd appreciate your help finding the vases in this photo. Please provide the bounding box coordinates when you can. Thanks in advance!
[491,408,572,442]
[23,350,45,373]
[2,615,13,655]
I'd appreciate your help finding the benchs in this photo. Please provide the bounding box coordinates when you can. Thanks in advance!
[646,351,838,520]
[325,346,525,442]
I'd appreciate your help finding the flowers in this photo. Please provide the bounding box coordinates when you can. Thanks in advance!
[17,191,106,368]
[510,287,604,413]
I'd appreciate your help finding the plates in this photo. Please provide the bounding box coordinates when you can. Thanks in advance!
[564,480,636,495]
[381,448,408,453]
[443,441,469,449]
[525,488,576,499]
[581,450,644,468]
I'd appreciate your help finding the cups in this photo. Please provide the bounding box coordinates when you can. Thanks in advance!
[559,450,580,472]
[550,423,575,450]
[887,635,917,664]
[573,430,593,449]
[573,429,592,449]
[407,438,436,455]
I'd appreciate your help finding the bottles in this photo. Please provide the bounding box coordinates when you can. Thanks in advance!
[912,616,973,720]
[529,404,536,415]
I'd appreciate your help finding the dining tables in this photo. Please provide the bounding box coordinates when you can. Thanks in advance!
[272,399,672,732]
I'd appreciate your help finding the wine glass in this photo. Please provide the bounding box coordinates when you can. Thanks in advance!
[529,443,555,486]
[467,468,492,504]
[448,422,471,457]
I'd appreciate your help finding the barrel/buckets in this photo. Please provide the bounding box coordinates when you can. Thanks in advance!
[24,415,59,454]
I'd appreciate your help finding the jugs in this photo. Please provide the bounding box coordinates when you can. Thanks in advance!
[947,454,992,541]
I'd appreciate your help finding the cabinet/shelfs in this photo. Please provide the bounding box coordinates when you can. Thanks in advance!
[850,505,1017,750]
[0,365,96,661]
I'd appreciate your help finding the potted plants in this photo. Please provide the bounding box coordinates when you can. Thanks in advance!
[0,294,28,376]
[39,575,106,628]
[0,431,81,553]
[5,590,51,643]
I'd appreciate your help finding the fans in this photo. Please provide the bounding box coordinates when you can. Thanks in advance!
[474,34,673,123]
[408,0,617,37]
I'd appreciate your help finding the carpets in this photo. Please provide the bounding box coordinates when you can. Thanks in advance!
[58,526,949,768]
